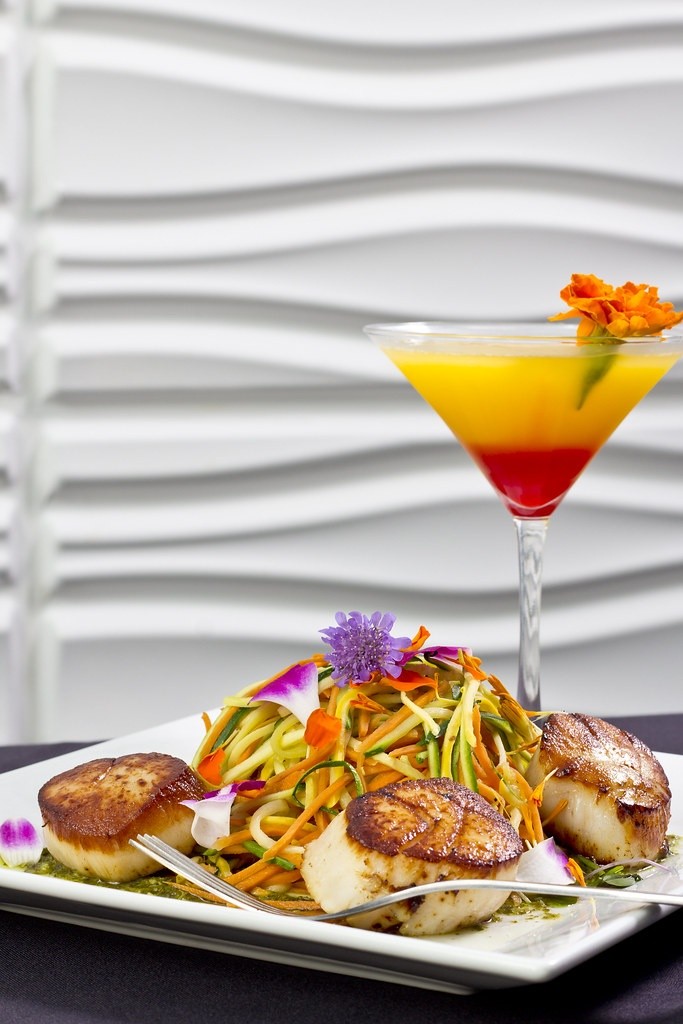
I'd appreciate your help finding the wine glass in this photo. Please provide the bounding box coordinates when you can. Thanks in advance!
[365,322,681,711]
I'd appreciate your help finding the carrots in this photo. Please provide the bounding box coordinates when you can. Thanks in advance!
[165,657,554,906]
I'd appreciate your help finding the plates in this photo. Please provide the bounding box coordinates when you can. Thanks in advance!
[1,712,682,995]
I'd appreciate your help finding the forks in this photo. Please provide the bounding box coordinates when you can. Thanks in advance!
[127,833,682,922]
